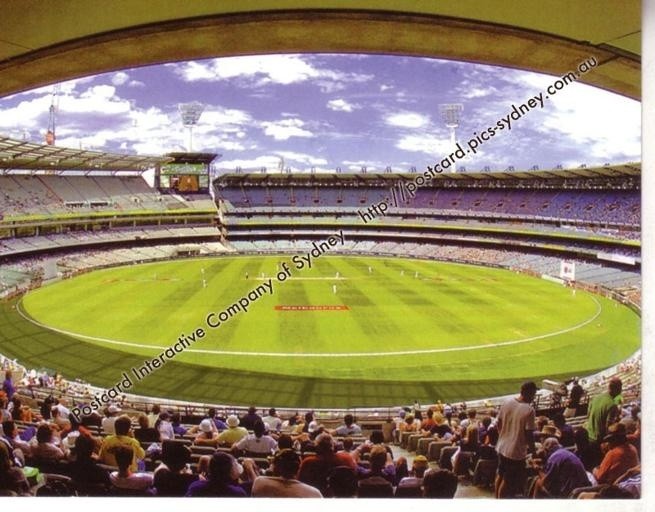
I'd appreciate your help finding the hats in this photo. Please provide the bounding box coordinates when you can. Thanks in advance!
[225,414,240,427]
[414,455,430,469]
[108,405,122,413]
[199,418,214,432]
[431,411,444,426]
[532,425,556,436]
[602,422,628,441]
[307,420,316,433]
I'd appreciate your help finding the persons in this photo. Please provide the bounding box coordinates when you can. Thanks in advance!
[1,174,642,497]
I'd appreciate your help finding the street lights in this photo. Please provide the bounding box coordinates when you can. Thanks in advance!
[436,101,465,175]
[178,100,207,152]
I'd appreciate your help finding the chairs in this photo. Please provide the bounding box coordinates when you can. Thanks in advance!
[0,330,641,499]
